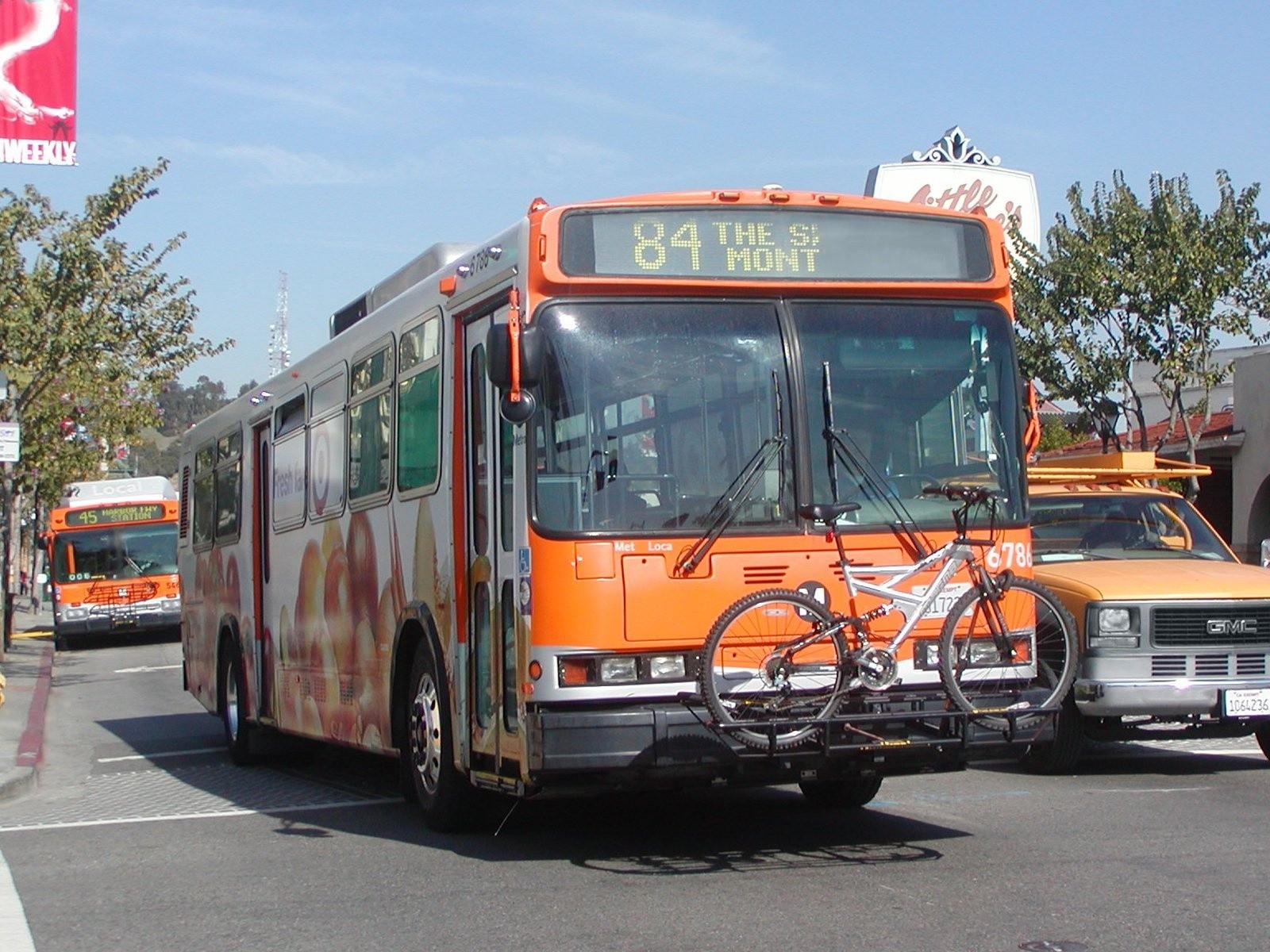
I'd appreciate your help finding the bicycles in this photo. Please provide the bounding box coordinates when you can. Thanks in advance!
[699,481,1080,754]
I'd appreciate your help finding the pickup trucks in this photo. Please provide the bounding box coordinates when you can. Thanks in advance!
[950,452,1270,762]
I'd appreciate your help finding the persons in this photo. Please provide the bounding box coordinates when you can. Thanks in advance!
[588,459,647,531]
[828,428,898,498]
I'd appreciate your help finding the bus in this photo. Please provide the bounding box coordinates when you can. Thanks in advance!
[177,188,1040,828]
[38,476,180,646]
